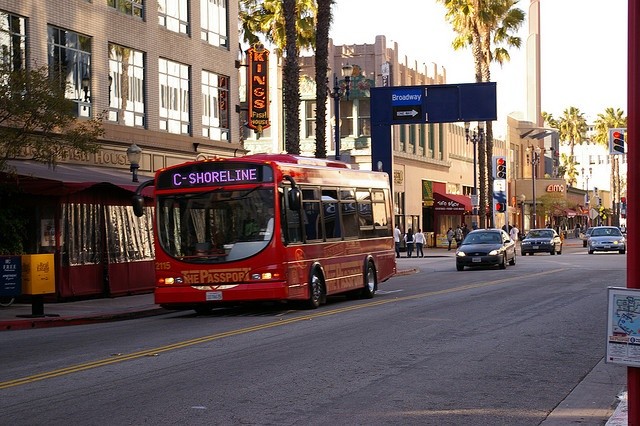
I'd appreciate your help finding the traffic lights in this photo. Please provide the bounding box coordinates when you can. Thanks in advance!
[495,203,505,212]
[610,129,623,156]
[497,158,506,180]
[621,197,626,203]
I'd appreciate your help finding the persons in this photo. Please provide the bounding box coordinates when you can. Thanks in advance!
[510,225,519,243]
[546,219,604,239]
[395,223,401,258]
[404,229,415,258]
[446,227,455,251]
[503,221,513,235]
[414,229,427,258]
[457,223,470,247]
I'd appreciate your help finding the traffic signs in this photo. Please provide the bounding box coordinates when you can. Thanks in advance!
[391,89,423,121]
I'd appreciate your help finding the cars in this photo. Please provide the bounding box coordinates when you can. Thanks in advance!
[583,227,597,248]
[521,229,562,256]
[588,226,626,254]
[456,229,516,271]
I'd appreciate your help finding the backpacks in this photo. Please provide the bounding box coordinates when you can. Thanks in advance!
[407,233,413,241]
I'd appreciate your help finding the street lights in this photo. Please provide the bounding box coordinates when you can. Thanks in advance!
[327,61,353,161]
[525,145,541,229]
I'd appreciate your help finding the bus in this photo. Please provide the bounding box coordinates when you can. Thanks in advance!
[133,155,396,314]
